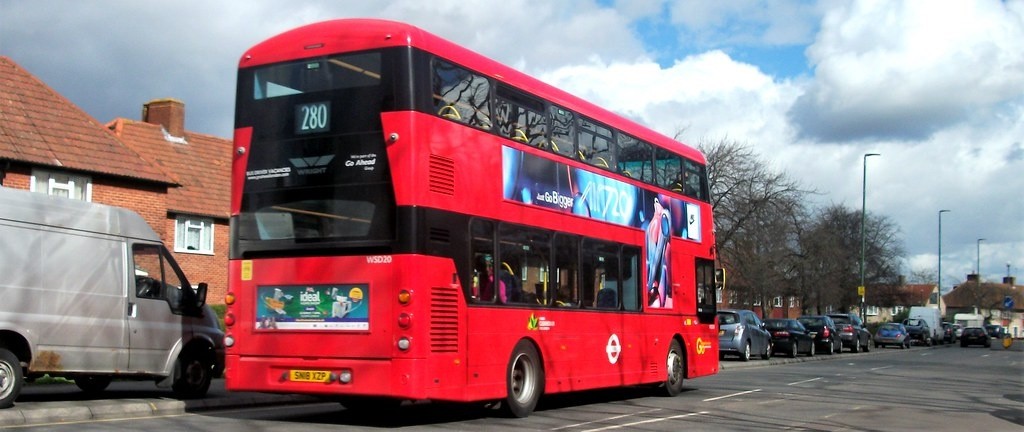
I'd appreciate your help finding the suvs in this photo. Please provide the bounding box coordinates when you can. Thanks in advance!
[902,318,933,345]
[824,313,873,354]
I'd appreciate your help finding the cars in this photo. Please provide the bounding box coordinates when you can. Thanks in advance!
[873,322,911,350]
[716,309,772,362]
[985,324,1005,339]
[797,315,842,354]
[942,322,964,344]
[960,326,992,348]
[762,318,815,358]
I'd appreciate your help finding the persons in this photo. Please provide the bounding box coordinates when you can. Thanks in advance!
[471,254,507,305]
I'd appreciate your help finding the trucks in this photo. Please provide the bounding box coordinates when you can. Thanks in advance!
[909,306,945,345]
[954,313,985,339]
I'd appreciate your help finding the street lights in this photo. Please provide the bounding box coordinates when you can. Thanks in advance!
[937,209,951,309]
[1005,261,1011,286]
[976,237,988,306]
[860,153,881,328]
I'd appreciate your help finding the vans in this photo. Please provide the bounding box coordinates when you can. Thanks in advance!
[0,186,225,413]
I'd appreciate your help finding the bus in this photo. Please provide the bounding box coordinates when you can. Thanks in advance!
[222,18,727,417]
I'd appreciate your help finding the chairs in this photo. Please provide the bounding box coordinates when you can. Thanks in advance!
[787,321,795,328]
[498,262,619,308]
[438,106,633,178]
[767,321,776,327]
